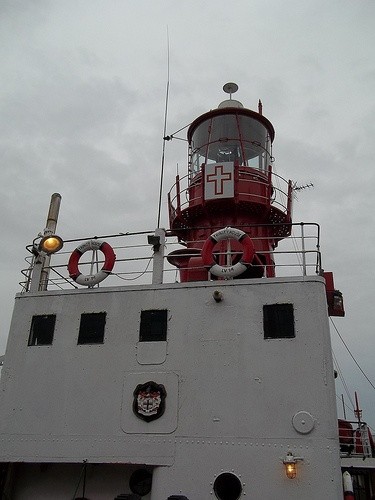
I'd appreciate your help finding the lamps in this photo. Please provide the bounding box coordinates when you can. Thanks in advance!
[284,451,296,480]
[31,227,64,264]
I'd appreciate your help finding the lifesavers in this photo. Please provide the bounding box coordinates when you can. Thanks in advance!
[343,470,354,500]
[356,426,373,453]
[201,228,255,277]
[68,240,116,286]
